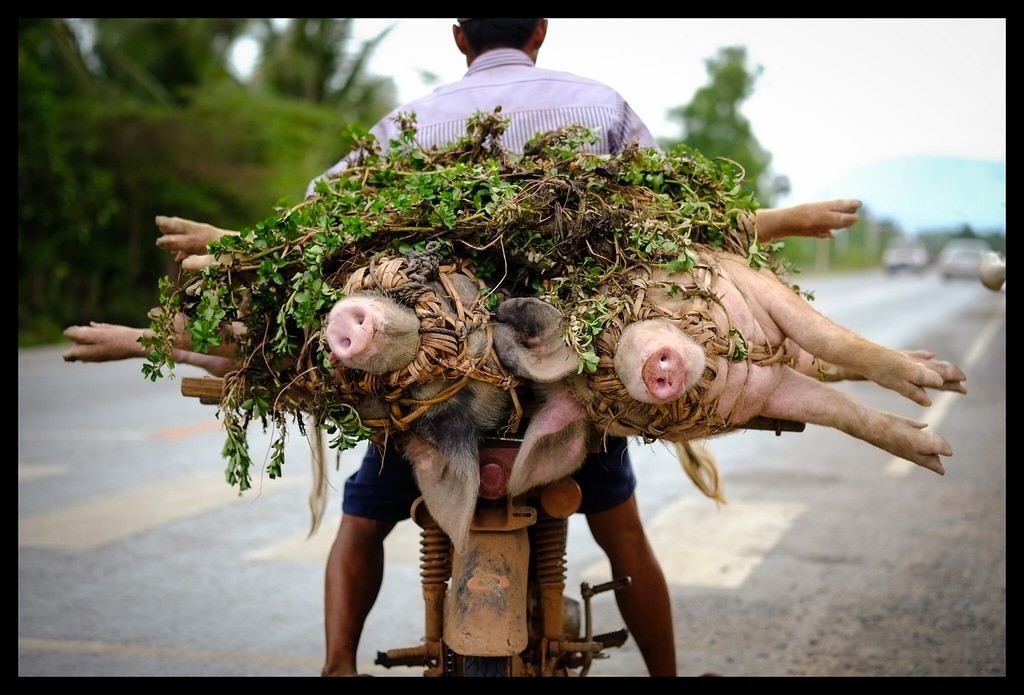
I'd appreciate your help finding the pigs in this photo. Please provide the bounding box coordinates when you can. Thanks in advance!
[59,197,972,555]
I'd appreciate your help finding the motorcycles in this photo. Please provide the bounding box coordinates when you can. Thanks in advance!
[372,425,632,676]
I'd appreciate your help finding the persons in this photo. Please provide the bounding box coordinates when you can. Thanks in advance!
[306,18,676,677]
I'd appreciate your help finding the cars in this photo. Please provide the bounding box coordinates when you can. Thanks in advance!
[882,238,927,274]
[938,237,992,281]
